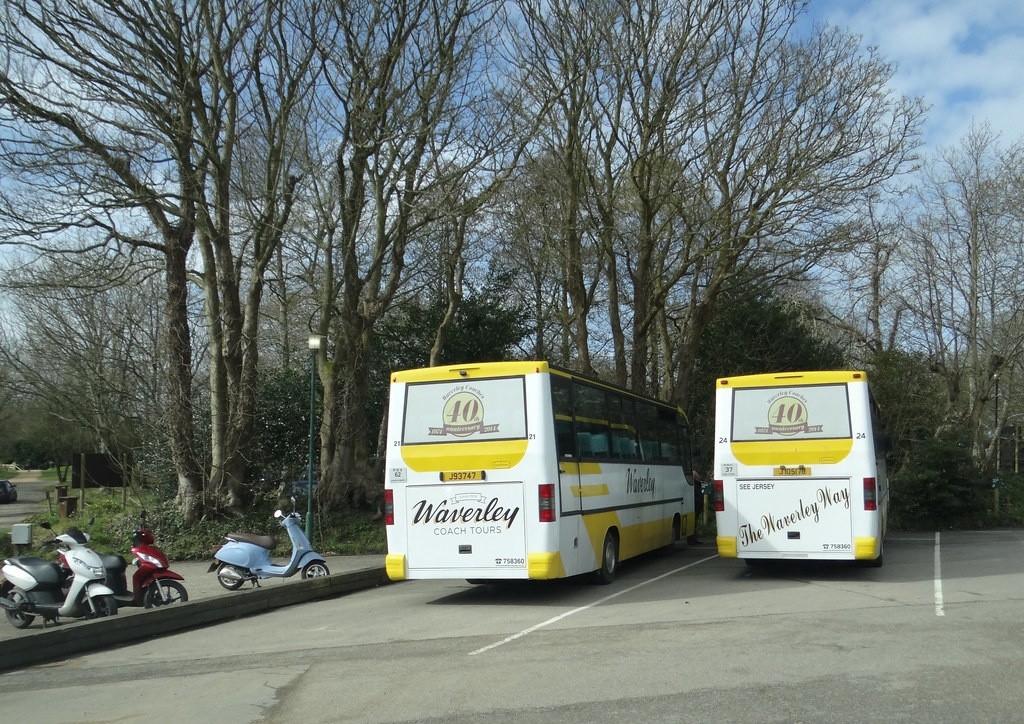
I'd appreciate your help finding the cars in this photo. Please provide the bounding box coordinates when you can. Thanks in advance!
[0,480,18,503]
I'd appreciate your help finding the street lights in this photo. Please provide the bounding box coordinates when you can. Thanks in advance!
[302,334,323,543]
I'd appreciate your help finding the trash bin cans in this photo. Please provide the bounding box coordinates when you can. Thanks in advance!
[54,484,69,498]
[58,496,80,517]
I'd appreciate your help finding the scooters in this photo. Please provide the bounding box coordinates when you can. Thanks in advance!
[207,496,331,589]
[0,509,188,628]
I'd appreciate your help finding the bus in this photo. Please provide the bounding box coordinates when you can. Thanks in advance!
[713,370,890,569]
[383,360,703,584]
